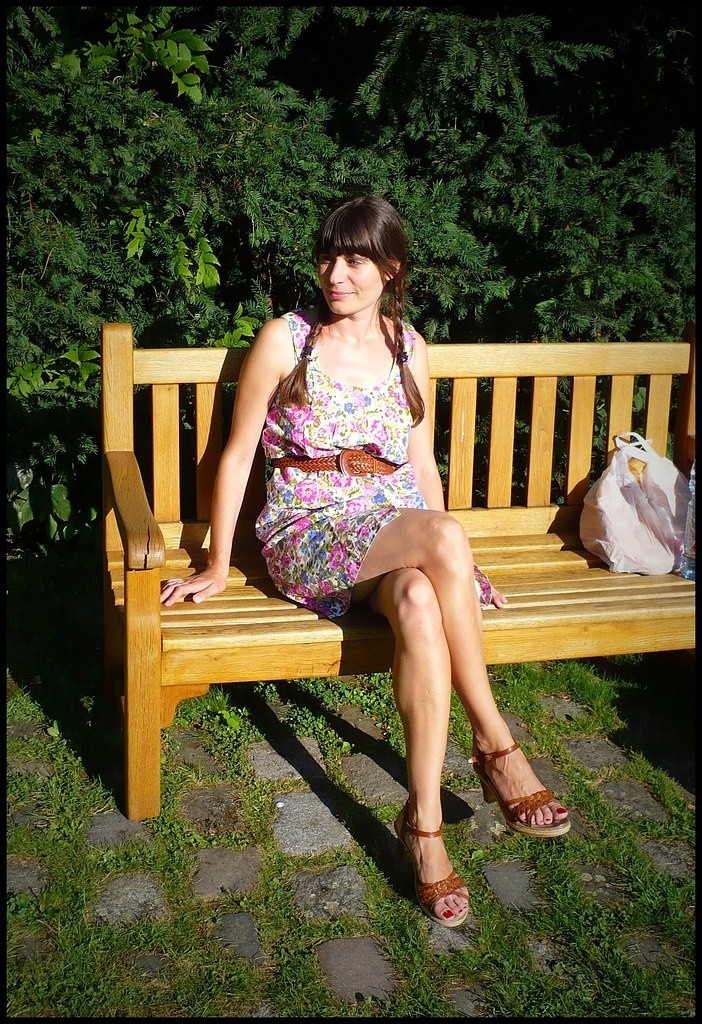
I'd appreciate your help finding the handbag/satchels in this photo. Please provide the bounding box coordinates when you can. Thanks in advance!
[580,432,692,576]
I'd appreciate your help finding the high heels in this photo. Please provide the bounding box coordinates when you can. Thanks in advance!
[468,743,572,837]
[394,798,469,927]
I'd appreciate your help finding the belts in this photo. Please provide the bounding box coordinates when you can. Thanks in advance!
[271,449,397,478]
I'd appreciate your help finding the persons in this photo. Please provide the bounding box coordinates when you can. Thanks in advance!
[159,196,571,929]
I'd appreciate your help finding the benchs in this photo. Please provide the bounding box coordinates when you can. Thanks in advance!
[99,323,695,821]
